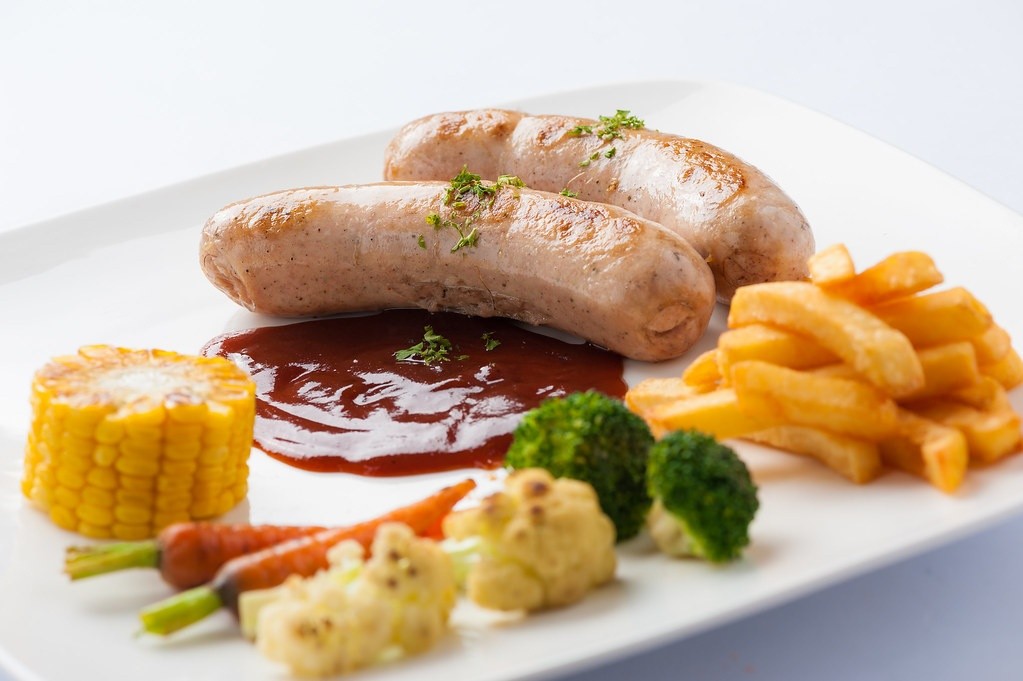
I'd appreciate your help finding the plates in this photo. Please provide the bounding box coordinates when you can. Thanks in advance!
[2,74,1023,681]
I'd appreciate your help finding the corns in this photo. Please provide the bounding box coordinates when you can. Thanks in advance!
[19,344,258,540]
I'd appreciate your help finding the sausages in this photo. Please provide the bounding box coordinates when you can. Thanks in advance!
[198,178,712,361]
[382,108,816,305]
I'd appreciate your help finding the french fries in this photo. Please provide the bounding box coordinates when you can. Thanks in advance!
[619,241,1023,494]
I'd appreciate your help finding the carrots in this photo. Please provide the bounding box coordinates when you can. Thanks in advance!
[158,474,477,614]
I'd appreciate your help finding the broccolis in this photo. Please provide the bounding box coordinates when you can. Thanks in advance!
[236,387,759,680]
